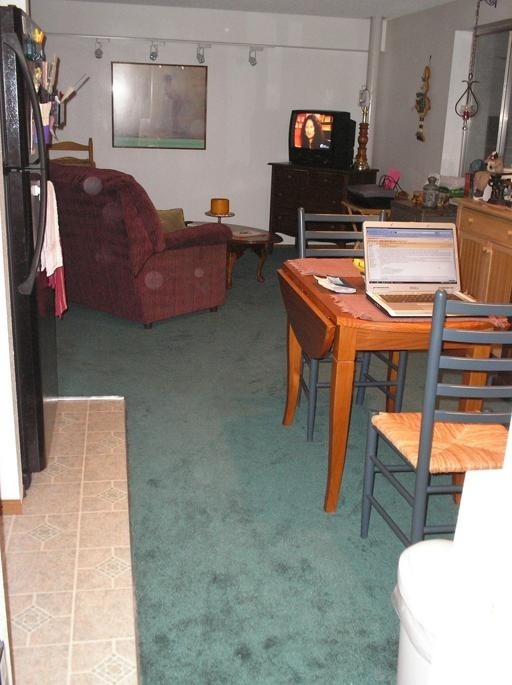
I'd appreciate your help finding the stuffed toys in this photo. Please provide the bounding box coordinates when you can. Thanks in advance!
[472,158,503,197]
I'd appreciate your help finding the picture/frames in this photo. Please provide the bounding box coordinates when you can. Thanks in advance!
[111,62,207,149]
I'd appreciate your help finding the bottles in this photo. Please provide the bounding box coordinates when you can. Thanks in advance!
[411,174,452,210]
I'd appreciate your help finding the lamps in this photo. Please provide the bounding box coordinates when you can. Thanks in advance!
[350,84,371,175]
[195,42,211,63]
[248,45,257,66]
[94,37,109,59]
[455,0,498,131]
[149,39,166,61]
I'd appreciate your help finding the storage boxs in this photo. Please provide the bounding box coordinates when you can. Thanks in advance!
[346,183,397,210]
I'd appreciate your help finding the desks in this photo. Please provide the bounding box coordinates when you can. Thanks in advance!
[276,256,512,513]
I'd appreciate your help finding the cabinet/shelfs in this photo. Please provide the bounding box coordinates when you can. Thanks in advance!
[389,199,457,226]
[450,196,512,358]
[267,161,379,256]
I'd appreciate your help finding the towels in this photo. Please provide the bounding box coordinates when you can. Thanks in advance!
[40,181,64,277]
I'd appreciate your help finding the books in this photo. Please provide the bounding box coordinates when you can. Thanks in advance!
[312,273,358,294]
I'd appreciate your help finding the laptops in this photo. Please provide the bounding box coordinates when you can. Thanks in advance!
[363,220,488,318]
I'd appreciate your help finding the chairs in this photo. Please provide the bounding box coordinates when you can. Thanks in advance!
[46,138,96,169]
[45,164,234,329]
[361,289,512,548]
[296,207,409,442]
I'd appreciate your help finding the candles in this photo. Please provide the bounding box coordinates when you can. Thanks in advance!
[211,198,230,215]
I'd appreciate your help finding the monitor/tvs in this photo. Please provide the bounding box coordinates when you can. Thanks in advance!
[289,110,356,169]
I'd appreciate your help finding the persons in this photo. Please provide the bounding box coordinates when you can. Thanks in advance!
[300,114,330,149]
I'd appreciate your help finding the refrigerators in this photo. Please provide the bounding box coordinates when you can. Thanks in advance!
[1,2,59,497]
